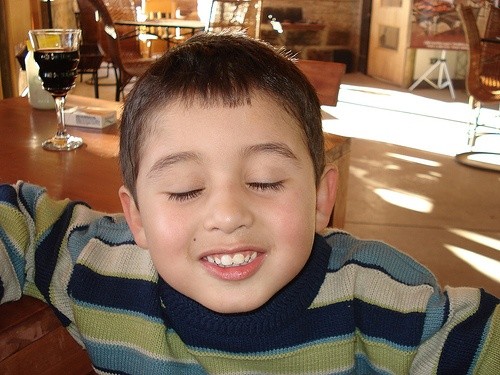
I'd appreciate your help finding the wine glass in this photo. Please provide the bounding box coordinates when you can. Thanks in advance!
[28,29,84,151]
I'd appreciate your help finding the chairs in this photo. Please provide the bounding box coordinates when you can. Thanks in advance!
[457,3,500,146]
[291,61,346,108]
[78,0,155,102]
[207,0,252,34]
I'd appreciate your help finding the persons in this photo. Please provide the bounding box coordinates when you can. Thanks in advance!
[0,30,500,375]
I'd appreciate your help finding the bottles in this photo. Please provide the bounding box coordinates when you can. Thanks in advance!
[24,36,62,109]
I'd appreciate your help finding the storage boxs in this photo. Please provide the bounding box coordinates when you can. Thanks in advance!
[58,106,116,129]
[259,9,357,72]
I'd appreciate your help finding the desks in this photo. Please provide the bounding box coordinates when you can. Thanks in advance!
[114,18,207,52]
[0,94,351,375]
[406,31,500,99]
